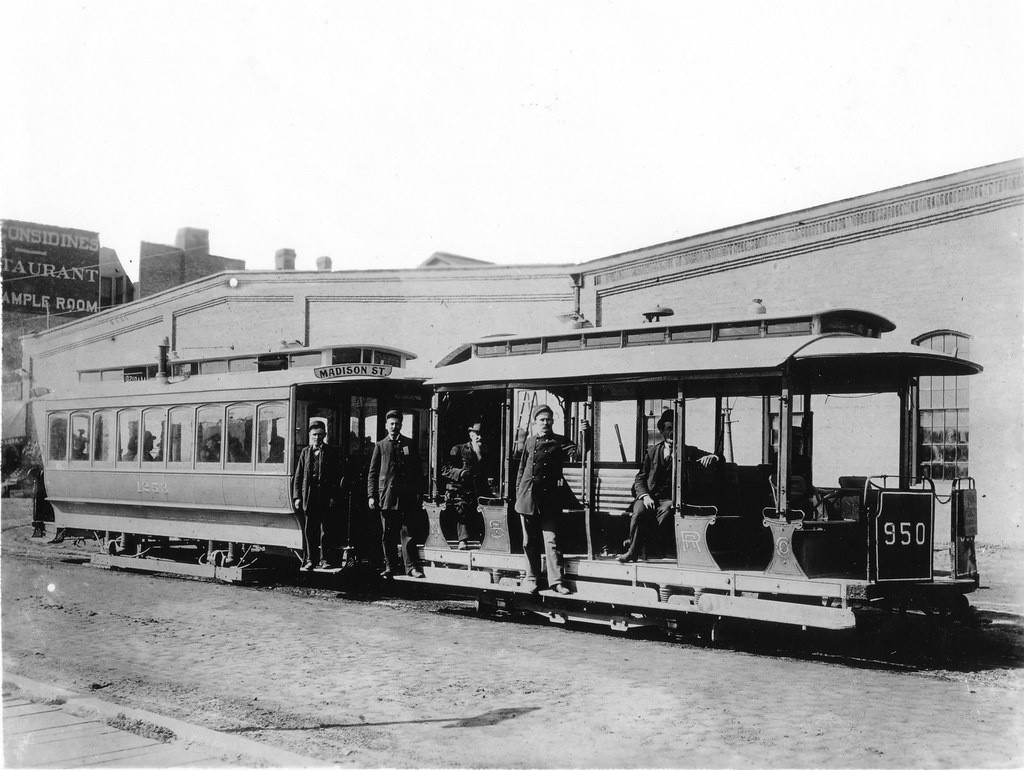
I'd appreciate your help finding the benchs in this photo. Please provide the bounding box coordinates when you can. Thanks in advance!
[414,454,645,554]
[675,456,861,579]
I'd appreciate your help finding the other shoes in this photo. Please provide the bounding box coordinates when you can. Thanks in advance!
[623,539,632,547]
[408,569,425,578]
[305,562,316,570]
[380,568,400,575]
[458,540,468,550]
[553,584,570,594]
[527,580,537,593]
[319,560,331,569]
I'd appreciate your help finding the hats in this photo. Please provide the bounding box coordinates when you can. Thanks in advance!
[533,404,553,420]
[469,422,485,431]
[309,421,325,431]
[386,410,403,421]
[657,409,677,432]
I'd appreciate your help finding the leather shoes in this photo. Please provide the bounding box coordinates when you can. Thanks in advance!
[616,552,637,563]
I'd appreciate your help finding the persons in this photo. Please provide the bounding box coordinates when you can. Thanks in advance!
[441,423,497,551]
[292,421,340,569]
[73,438,90,460]
[514,404,590,595]
[918,426,962,479]
[122,431,156,461]
[616,410,726,562]
[367,411,425,578]
[199,438,219,462]
[230,441,249,463]
[263,437,284,464]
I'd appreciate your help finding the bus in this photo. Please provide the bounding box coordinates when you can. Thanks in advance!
[29,326,983,630]
